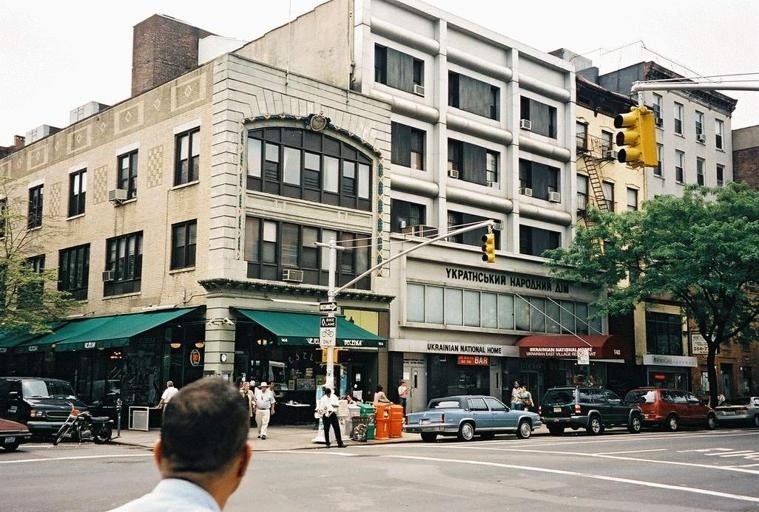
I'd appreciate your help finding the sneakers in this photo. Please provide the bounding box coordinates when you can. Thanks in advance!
[338,443,348,448]
[326,443,331,448]
[257,434,266,440]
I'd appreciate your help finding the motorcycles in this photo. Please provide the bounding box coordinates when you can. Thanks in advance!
[48,396,114,444]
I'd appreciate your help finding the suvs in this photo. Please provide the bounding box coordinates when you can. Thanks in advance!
[0,375,88,442]
[621,386,719,433]
[538,386,643,436]
[74,379,121,405]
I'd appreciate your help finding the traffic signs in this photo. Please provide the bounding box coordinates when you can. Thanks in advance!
[318,303,337,310]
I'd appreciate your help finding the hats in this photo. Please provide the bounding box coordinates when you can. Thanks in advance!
[258,382,270,388]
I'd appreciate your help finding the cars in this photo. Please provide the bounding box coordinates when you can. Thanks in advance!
[404,393,545,441]
[0,416,32,453]
[711,396,759,428]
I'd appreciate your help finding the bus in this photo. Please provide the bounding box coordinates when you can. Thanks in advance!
[250,360,289,391]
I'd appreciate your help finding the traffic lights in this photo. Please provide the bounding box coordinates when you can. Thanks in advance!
[610,110,641,164]
[481,234,493,264]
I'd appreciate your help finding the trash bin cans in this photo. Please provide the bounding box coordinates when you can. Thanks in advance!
[357,403,375,440]
[376,403,404,441]
[350,417,368,439]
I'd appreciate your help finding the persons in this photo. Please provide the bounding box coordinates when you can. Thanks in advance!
[156,381,178,415]
[398,380,411,408]
[373,383,389,403]
[317,388,347,448]
[239,380,276,439]
[103,375,251,512]
[717,392,725,406]
[347,394,355,404]
[511,381,523,410]
[521,385,534,411]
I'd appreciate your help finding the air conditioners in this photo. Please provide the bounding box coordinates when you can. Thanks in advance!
[109,189,128,201]
[548,191,560,203]
[403,224,438,239]
[448,170,459,178]
[413,84,424,97]
[698,134,706,142]
[607,150,618,159]
[656,117,663,126]
[488,181,499,190]
[522,188,532,197]
[282,268,304,283]
[520,119,531,131]
[102,271,114,282]
